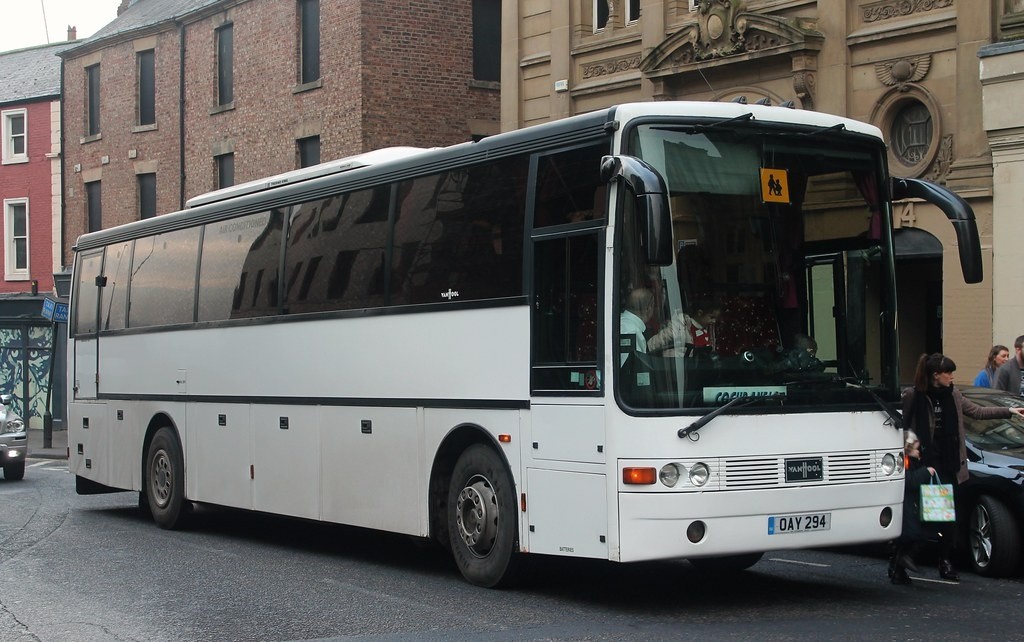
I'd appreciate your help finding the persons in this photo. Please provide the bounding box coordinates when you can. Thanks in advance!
[534,190,827,397]
[887,427,938,587]
[973,345,1010,389]
[899,352,1024,587]
[991,334,1024,398]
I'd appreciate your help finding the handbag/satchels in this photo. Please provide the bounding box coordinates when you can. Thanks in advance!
[920,468,957,523]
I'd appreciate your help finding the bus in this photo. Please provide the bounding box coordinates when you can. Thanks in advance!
[67,98,983,588]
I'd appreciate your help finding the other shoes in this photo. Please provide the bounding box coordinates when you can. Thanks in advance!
[940,560,960,582]
[887,563,912,584]
[903,555,920,573]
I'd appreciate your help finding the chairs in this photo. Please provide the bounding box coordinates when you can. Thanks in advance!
[574,278,786,362]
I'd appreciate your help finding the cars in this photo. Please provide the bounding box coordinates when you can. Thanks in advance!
[818,381,1024,578]
[0,394,27,482]
